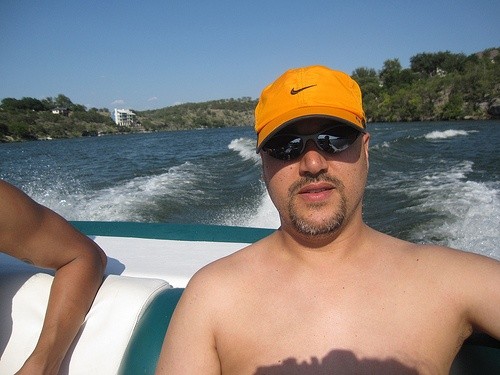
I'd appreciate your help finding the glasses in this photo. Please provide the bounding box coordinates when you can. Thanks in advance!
[261,123,362,161]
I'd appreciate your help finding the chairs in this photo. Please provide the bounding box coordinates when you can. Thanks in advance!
[1,270,187,375]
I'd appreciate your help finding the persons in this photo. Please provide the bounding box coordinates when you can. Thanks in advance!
[155,66,500,375]
[0,179,107,375]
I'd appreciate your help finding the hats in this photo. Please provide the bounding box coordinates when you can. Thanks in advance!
[254,65,367,154]
